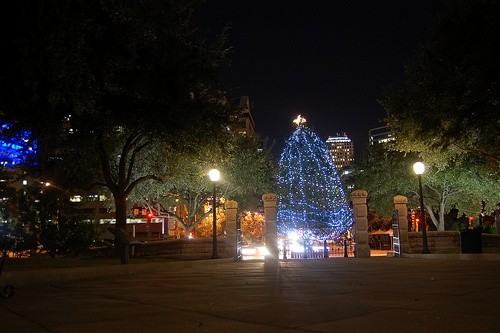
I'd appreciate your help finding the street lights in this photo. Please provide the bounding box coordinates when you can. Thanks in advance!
[412,162,432,254]
[208,168,221,259]
[480,200,487,226]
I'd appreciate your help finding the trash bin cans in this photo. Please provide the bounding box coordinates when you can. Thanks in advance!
[460,228,482,254]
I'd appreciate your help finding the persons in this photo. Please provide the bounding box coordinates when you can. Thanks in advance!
[237,229,244,260]
[106,226,131,265]
